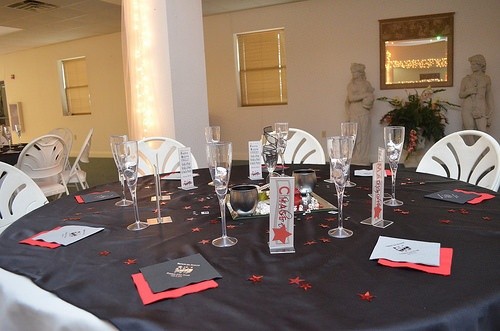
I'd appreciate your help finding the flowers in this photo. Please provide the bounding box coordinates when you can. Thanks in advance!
[376,85,460,161]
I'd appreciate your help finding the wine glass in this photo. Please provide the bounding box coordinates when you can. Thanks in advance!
[14,125,25,148]
[275,122,289,177]
[323,122,358,187]
[327,134,355,238]
[206,141,238,248]
[205,125,221,186]
[109,135,150,231]
[0,125,15,152]
[263,126,281,190]
[384,126,405,206]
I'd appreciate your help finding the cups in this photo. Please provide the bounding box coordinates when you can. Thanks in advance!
[230,185,258,217]
[292,169,317,194]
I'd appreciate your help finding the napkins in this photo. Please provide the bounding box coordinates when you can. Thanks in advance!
[161,174,199,181]
[369,237,440,266]
[354,169,373,177]
[34,225,104,247]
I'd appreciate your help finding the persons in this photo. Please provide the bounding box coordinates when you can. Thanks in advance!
[460,54,494,162]
[346,63,375,165]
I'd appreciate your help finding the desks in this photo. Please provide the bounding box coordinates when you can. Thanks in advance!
[1,163,500,331]
[0,143,28,165]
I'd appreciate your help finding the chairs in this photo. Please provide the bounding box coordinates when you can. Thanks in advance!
[0,127,93,234]
[117,137,198,180]
[261,128,325,164]
[415,129,500,192]
